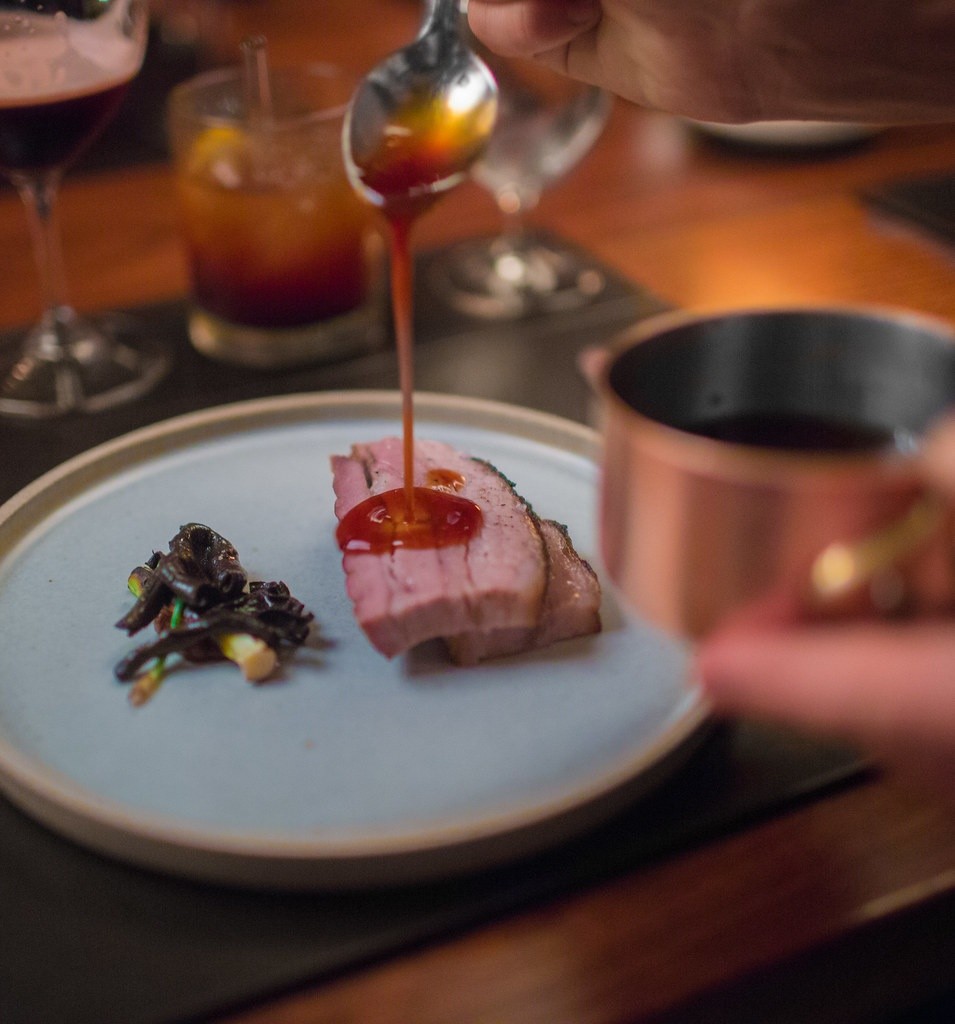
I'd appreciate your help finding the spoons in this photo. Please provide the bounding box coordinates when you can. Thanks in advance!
[341,0,500,208]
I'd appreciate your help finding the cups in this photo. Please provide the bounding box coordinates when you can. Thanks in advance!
[165,62,379,357]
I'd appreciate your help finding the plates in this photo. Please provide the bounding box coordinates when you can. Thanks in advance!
[695,121,898,156]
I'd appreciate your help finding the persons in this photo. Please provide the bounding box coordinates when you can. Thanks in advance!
[465,0,955,763]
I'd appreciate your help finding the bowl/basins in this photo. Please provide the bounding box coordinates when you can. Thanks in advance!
[588,301,955,639]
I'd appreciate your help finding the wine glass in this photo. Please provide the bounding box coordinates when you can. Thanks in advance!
[1,1,174,417]
[428,4,619,324]
